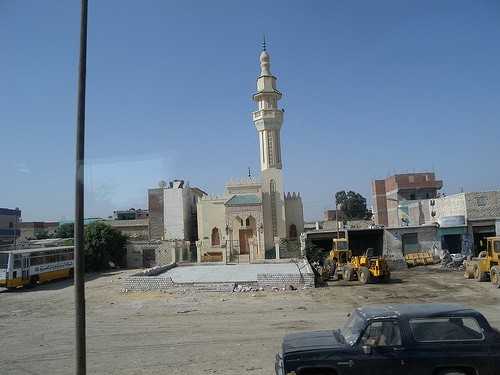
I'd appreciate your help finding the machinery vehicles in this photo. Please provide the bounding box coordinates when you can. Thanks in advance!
[463,236,499,289]
[323,238,391,285]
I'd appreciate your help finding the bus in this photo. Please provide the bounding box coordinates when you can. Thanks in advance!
[0,246,74,290]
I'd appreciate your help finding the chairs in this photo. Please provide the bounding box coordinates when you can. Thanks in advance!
[390,325,401,345]
[377,322,392,345]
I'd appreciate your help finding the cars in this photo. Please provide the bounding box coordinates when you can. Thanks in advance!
[274,302,499,375]
